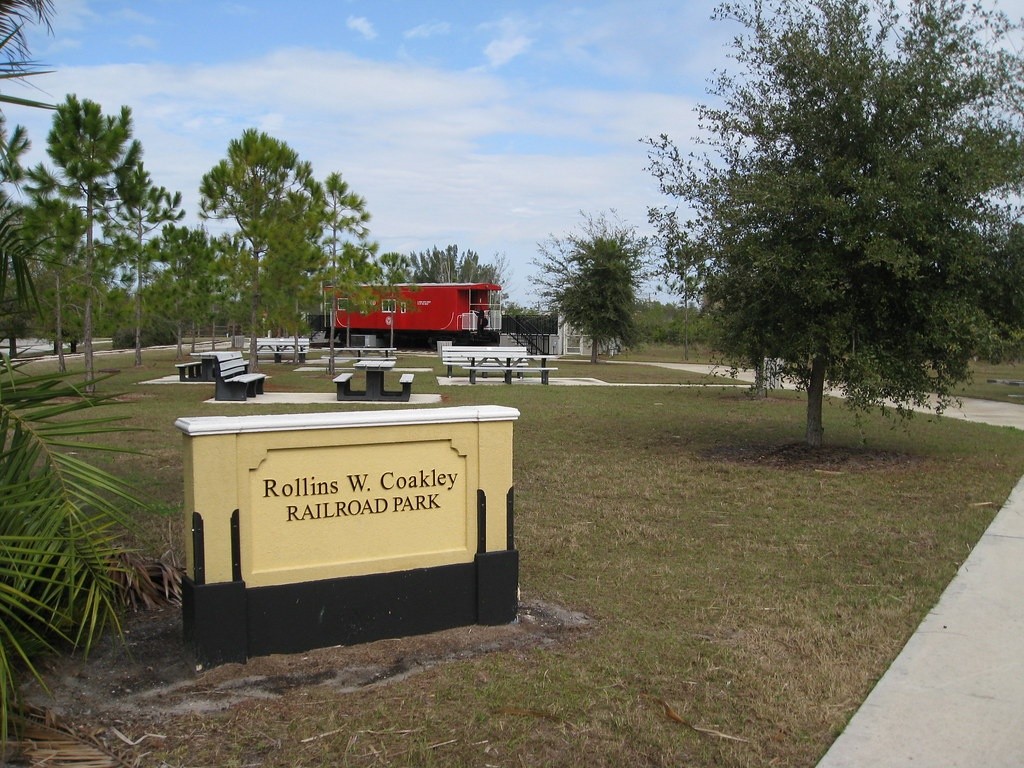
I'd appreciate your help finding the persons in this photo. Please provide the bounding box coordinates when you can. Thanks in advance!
[474,305,487,336]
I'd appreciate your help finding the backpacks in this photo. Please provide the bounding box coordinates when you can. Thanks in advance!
[480,318,488,327]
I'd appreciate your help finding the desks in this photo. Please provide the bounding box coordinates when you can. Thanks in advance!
[352,361,396,400]
[190,352,216,382]
[461,355,559,385]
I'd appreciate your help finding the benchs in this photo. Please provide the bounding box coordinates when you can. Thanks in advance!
[399,373,414,402]
[442,346,529,378]
[215,352,266,401]
[333,373,353,401]
[242,338,310,363]
[459,363,558,385]
[175,362,202,382]
[321,348,397,362]
